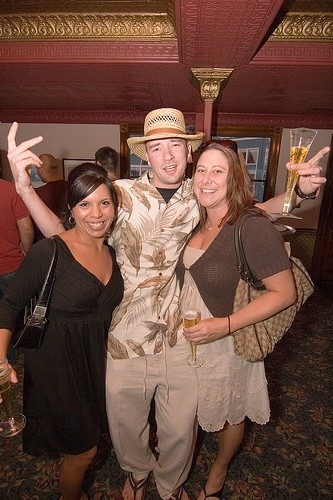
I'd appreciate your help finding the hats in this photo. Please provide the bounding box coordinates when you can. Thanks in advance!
[127,108,203,161]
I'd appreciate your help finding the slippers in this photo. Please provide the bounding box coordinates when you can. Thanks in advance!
[195,473,227,500]
[151,442,160,455]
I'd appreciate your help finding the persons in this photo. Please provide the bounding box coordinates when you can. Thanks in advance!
[7,109,330,500]
[152,141,297,500]
[0,162,124,500]
[0,146,123,363]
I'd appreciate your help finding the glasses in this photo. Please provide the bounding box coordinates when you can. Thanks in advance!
[205,140,241,167]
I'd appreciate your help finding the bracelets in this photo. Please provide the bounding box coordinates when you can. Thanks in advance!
[227,314,231,336]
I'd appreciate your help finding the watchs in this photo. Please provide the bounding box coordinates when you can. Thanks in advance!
[294,183,320,200]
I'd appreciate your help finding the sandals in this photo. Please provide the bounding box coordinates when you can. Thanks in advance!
[168,485,190,500]
[121,472,150,500]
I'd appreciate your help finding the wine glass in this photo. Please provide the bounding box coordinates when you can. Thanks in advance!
[269,127,318,220]
[183,309,203,367]
[0,357,27,438]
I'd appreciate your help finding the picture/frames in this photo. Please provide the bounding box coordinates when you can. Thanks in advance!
[119,123,283,203]
[62,158,96,182]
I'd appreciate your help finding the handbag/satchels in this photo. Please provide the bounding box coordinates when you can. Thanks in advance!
[11,238,59,349]
[232,210,314,363]
